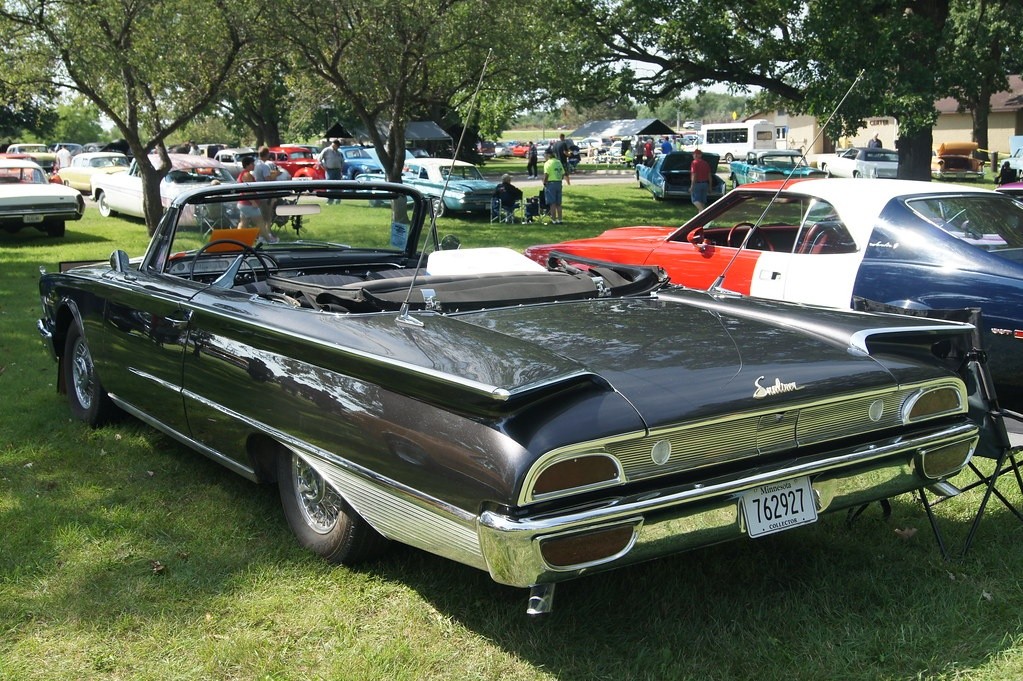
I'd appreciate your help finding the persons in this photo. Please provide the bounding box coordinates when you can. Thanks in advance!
[625,136,681,168]
[177,138,199,155]
[234,148,279,244]
[996,162,1016,185]
[552,134,581,175]
[56,144,71,168]
[543,148,570,224]
[49,169,63,184]
[317,140,344,205]
[154,146,158,154]
[689,150,712,213]
[208,179,234,228]
[493,173,523,210]
[866,133,883,155]
[527,140,538,179]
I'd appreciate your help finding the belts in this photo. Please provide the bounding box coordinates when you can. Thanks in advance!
[696,180,709,183]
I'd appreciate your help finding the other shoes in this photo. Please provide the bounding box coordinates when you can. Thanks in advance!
[557,219,563,223]
[552,220,556,223]
[268,237,280,244]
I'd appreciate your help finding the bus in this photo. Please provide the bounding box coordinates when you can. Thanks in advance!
[681,119,777,164]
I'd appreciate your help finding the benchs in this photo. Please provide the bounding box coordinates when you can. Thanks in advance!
[288,267,429,287]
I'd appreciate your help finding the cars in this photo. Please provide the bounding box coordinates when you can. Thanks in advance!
[522,176,1023,417]
[816,144,899,180]
[931,140,984,180]
[474,134,702,165]
[634,149,726,202]
[940,179,1023,246]
[0,141,382,203]
[353,147,499,218]
[0,159,87,237]
[1000,136,1023,182]
[89,152,240,231]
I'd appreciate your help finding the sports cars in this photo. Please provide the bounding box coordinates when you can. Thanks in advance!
[34,177,982,615]
[728,148,826,199]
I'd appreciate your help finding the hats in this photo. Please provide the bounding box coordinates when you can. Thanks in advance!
[545,148,554,154]
[693,149,702,154]
[333,140,342,147]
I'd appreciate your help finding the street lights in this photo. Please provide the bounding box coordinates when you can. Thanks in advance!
[320,102,331,149]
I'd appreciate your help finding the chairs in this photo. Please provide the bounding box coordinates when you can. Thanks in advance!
[843,294,1023,565]
[881,156,890,160]
[797,221,856,254]
[490,188,551,224]
[194,190,302,243]
